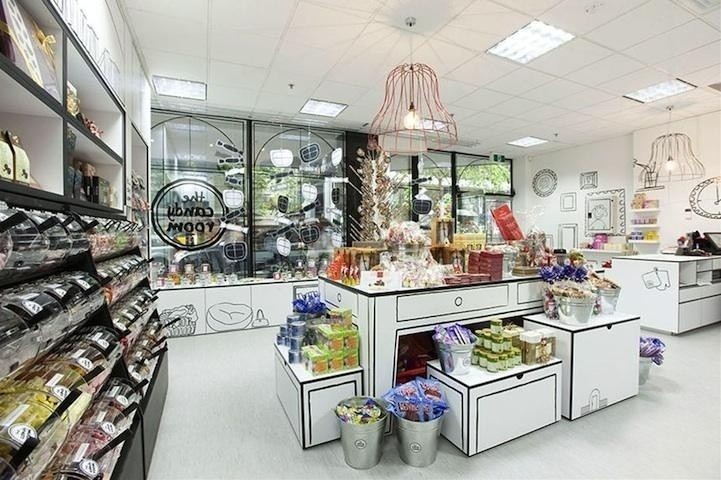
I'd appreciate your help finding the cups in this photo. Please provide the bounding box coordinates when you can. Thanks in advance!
[644,172,658,188]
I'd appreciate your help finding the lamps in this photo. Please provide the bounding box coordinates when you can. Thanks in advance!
[368,15,462,157]
[637,102,706,192]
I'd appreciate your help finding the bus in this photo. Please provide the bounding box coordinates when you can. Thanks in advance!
[301,175,510,237]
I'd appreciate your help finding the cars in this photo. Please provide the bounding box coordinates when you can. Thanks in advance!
[146,213,330,277]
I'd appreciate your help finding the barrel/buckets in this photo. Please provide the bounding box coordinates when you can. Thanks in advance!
[396,413,444,468]
[330,397,389,470]
[597,286,622,314]
[559,297,598,326]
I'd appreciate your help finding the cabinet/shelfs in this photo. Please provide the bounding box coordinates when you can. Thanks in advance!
[611,257,720,337]
[627,201,661,245]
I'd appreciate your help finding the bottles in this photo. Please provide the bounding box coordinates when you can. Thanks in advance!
[472,318,523,373]
[277,315,307,364]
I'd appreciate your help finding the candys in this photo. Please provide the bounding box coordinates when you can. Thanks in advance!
[356,140,394,242]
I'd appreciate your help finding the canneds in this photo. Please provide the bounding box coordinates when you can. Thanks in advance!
[470,318,523,373]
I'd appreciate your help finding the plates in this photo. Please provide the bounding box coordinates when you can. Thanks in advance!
[637,185,664,192]
